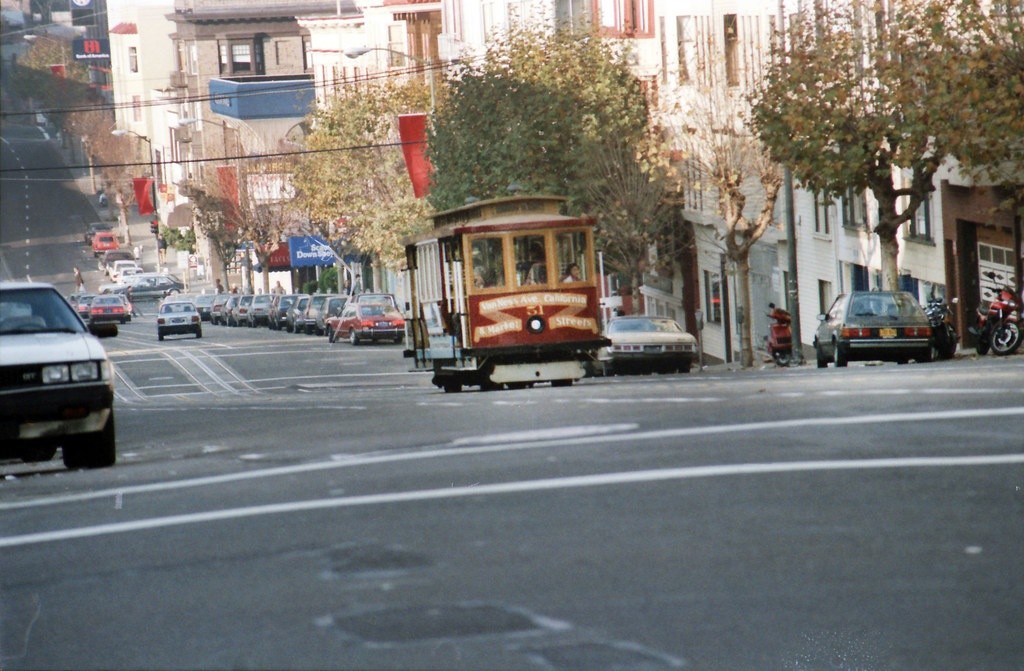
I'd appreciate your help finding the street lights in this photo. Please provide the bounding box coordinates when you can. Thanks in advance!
[344,46,438,121]
[111,129,162,272]
[22,34,67,78]
[179,116,242,240]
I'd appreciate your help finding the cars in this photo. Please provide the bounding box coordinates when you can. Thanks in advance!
[158,302,204,342]
[83,222,188,301]
[87,294,127,325]
[68,291,96,319]
[163,292,214,320]
[221,295,241,325]
[267,293,309,330]
[286,296,310,334]
[766,302,796,367]
[304,294,342,335]
[602,315,699,376]
[250,294,274,328]
[314,296,352,337]
[811,289,937,367]
[342,293,400,315]
[324,302,406,346]
[210,293,231,324]
[233,294,255,327]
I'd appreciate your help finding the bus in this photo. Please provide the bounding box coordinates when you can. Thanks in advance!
[398,192,613,392]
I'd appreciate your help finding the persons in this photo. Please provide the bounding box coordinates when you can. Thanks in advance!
[216,279,223,294]
[73,268,84,292]
[232,283,254,294]
[125,286,136,317]
[475,243,546,287]
[158,234,167,264]
[147,279,153,286]
[563,263,579,283]
[343,274,361,295]
[272,281,284,294]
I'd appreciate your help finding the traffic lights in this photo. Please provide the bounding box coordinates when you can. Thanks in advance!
[150,220,159,234]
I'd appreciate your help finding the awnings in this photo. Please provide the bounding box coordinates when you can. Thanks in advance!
[253,234,335,272]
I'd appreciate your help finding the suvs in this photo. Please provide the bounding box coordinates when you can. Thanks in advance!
[1,282,119,467]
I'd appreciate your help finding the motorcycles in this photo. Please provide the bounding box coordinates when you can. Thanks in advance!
[976,270,1024,355]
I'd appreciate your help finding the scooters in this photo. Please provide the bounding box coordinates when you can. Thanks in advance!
[920,294,962,360]
